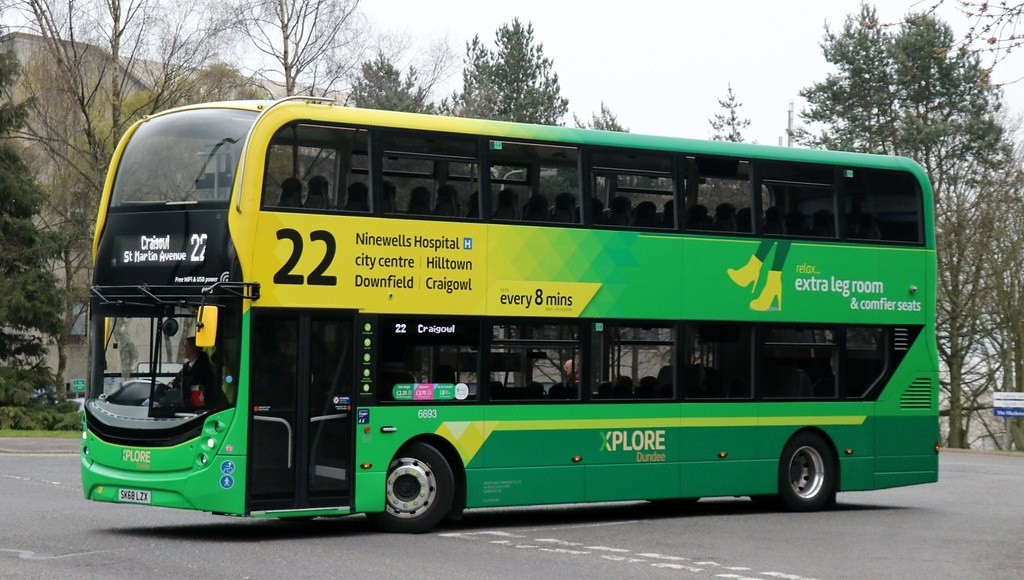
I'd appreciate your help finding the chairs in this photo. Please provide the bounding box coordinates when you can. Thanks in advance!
[376,362,884,399]
[277,175,881,244]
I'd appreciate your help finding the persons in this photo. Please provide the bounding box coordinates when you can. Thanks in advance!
[168,338,218,407]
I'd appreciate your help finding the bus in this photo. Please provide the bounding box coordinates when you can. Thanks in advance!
[81,98,941,534]
